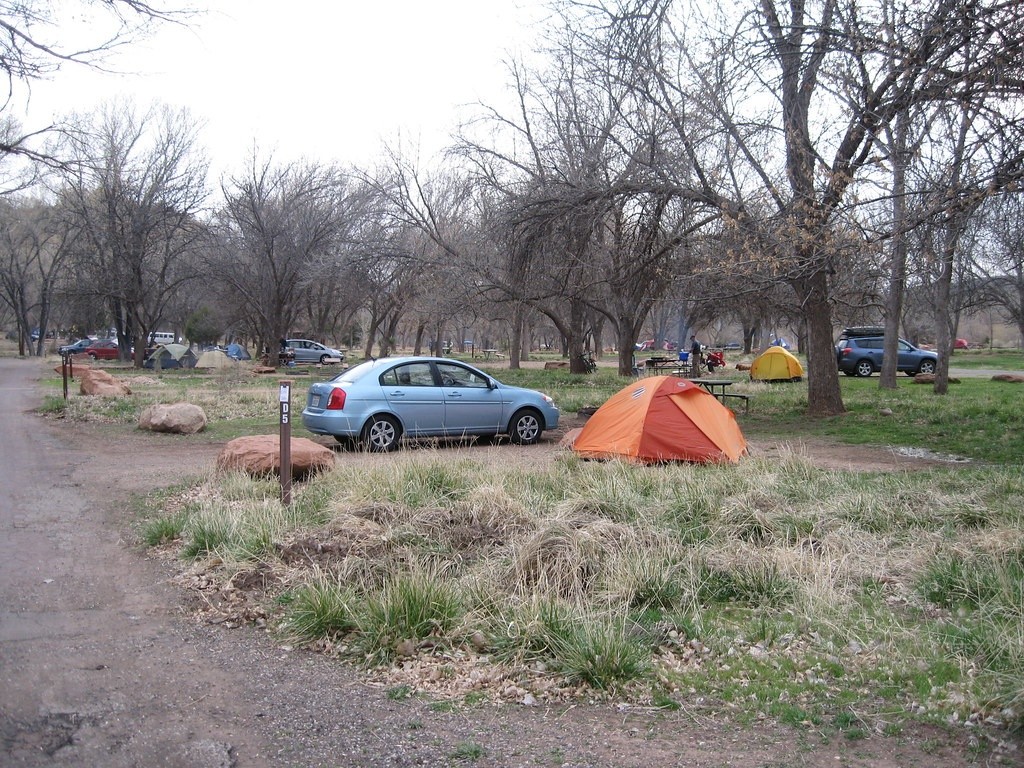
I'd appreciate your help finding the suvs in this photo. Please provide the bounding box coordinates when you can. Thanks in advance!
[834,326,938,376]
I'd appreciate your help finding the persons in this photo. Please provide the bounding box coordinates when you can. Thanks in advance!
[688,335,701,379]
[265,337,288,356]
[703,351,722,374]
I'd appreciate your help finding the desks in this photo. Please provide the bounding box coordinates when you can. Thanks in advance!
[482,349,498,360]
[653,358,687,375]
[323,358,342,375]
[686,379,732,400]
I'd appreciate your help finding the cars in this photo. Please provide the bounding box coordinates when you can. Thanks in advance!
[264,339,344,363]
[540,344,551,349]
[633,340,674,351]
[301,356,561,453]
[86,341,135,360]
[464,340,472,344]
[429,341,453,354]
[58,340,93,356]
[723,343,743,350]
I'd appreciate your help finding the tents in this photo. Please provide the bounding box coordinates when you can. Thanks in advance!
[572,375,750,470]
[146,343,251,371]
[750,337,804,382]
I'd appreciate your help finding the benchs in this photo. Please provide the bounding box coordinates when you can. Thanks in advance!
[475,355,484,359]
[714,393,752,406]
[648,366,691,378]
[496,354,505,360]
[343,362,348,375]
[316,363,322,376]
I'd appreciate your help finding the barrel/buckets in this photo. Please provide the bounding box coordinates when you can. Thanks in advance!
[679,349,689,361]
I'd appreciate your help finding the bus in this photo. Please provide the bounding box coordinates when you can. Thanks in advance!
[148,332,174,346]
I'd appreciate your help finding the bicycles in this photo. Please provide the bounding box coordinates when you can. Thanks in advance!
[577,351,596,374]
